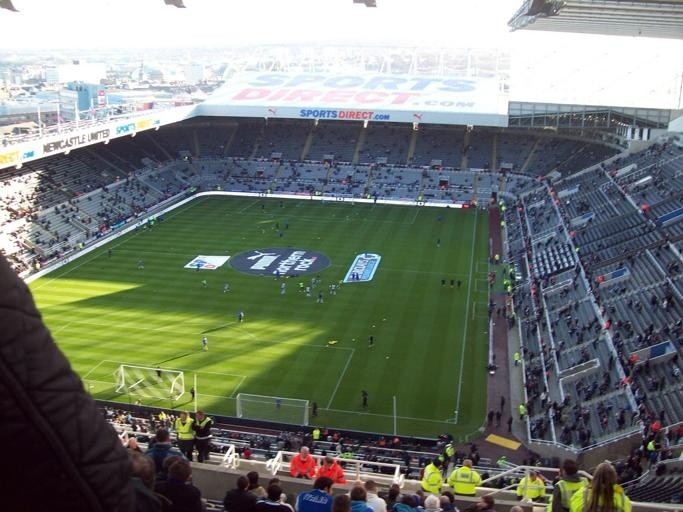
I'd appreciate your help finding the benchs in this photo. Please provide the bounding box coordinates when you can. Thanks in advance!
[198,108,682,205]
[100,402,490,512]
[0,113,198,280]
[488,205,683,512]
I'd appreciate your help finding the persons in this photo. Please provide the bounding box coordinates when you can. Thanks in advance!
[311,402,318,415]
[190,387,194,400]
[275,396,281,407]
[440,279,462,288]
[200,280,208,288]
[239,312,244,323]
[107,408,493,512]
[199,116,485,205]
[202,337,208,346]
[262,200,283,211]
[135,399,141,404]
[155,367,161,376]
[362,391,368,406]
[1,102,199,275]
[485,101,682,512]
[436,238,441,247]
[368,336,374,346]
[276,221,288,236]
[0,256,167,509]
[436,213,442,222]
[275,269,359,305]
[224,281,232,294]
